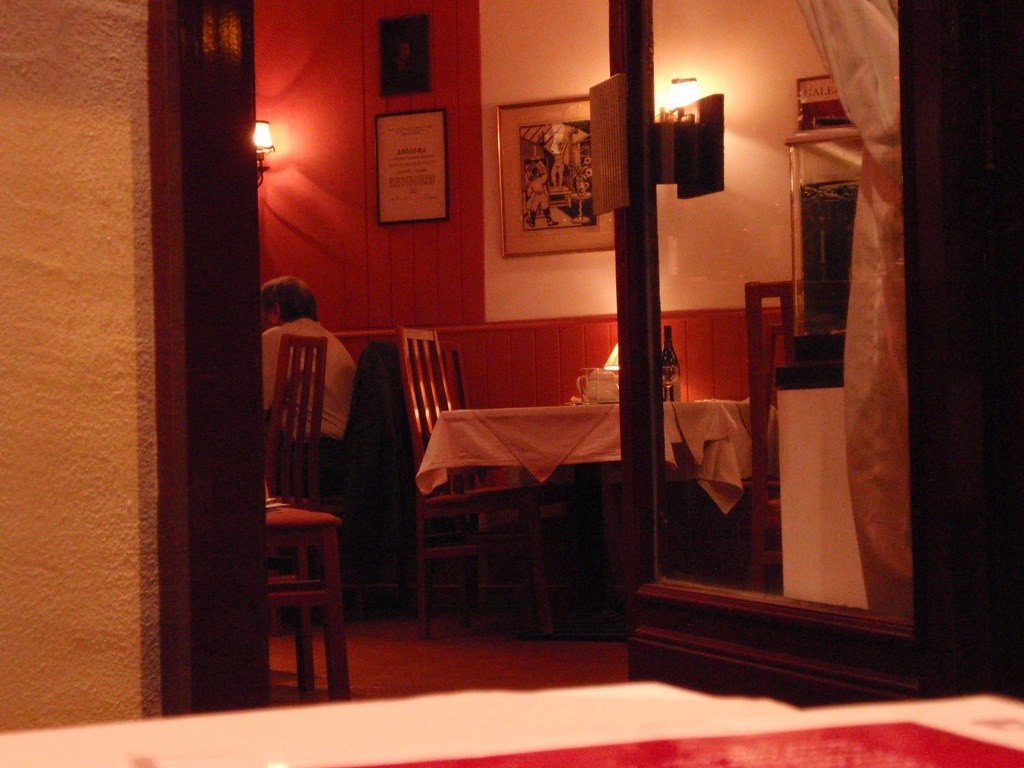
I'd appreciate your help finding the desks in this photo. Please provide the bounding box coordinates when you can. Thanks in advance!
[416,397,775,635]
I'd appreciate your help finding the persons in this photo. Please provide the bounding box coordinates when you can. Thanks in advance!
[261,277,357,636]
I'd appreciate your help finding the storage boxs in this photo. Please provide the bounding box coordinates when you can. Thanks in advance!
[784,125,865,365]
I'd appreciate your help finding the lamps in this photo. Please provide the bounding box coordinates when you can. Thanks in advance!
[253,120,275,185]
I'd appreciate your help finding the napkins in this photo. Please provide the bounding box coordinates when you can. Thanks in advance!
[736,402,776,437]
[471,404,615,483]
[671,401,738,465]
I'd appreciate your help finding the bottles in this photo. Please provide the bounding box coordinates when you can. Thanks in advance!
[661,325,680,402]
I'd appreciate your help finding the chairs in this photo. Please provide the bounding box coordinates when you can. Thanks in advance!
[259,282,809,703]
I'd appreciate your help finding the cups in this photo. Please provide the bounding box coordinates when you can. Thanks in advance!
[575,367,619,403]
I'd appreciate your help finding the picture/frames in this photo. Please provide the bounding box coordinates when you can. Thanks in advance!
[496,96,616,259]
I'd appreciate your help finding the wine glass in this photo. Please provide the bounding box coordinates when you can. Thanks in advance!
[661,366,679,402]
